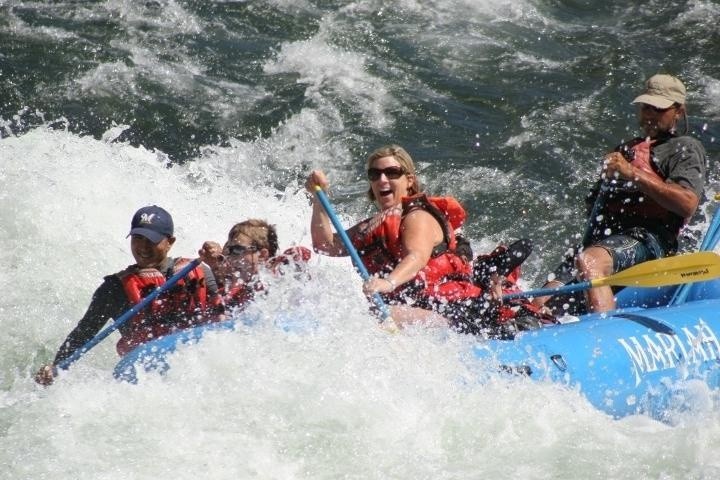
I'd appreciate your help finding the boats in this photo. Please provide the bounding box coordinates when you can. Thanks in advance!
[113,201,719,423]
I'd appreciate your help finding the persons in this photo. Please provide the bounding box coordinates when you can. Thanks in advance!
[527,74,709,315]
[223,219,311,310]
[34,205,227,387]
[306,146,473,330]
[457,235,561,341]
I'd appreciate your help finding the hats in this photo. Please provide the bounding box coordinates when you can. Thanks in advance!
[125,205,174,244]
[632,74,687,109]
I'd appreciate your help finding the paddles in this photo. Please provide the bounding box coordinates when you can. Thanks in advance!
[502,251,719,303]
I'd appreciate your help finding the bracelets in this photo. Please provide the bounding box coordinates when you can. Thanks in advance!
[386,280,395,294]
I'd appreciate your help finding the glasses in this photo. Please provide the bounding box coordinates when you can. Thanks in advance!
[226,245,259,256]
[367,166,409,181]
